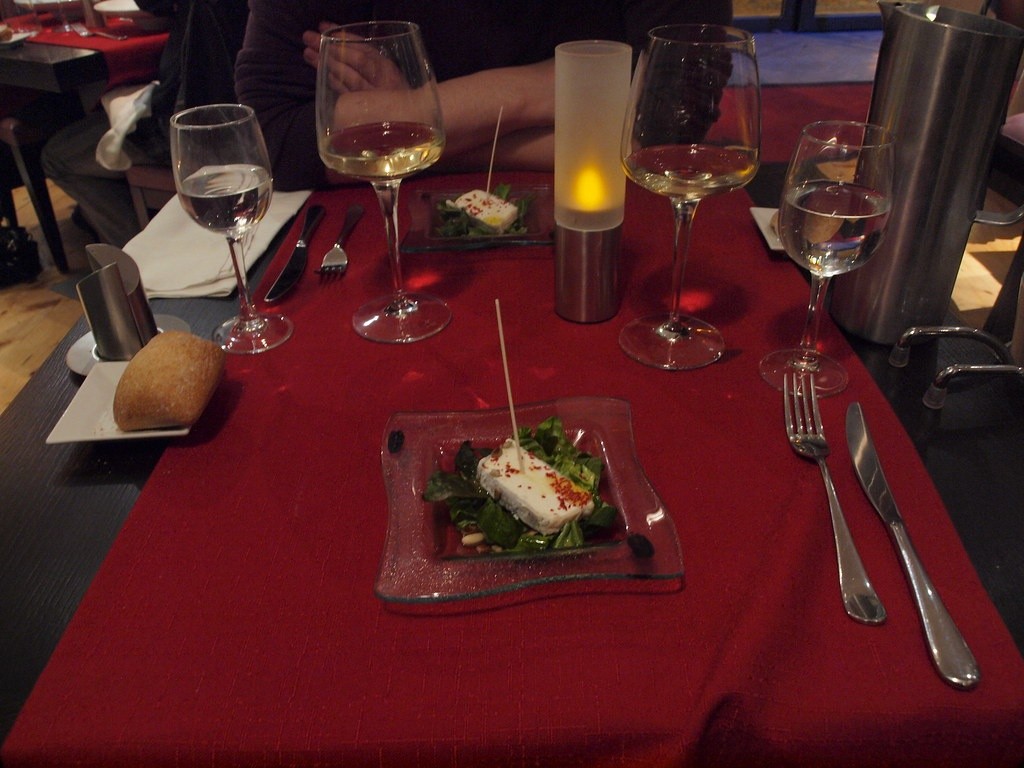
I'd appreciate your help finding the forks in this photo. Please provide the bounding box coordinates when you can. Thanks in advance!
[315,202,363,272]
[784,373,886,624]
[71,22,128,40]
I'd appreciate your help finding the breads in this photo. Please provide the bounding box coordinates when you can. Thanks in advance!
[113,329,226,431]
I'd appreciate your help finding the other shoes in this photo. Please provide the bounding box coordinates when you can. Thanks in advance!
[70,204,94,229]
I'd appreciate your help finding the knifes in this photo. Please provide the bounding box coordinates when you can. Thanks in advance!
[265,203,322,303]
[845,401,978,688]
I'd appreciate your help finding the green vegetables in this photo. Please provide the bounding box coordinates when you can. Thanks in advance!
[434,182,532,238]
[422,417,616,555]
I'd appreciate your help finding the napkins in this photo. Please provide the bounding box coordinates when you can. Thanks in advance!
[123,190,315,300]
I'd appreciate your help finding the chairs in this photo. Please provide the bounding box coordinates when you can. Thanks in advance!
[127,164,178,231]
[887,194,1023,658]
[0,0,76,275]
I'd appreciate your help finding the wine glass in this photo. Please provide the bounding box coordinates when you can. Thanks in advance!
[314,21,451,343]
[619,23,760,370]
[169,104,294,352]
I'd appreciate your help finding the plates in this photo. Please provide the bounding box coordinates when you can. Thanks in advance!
[1,32,31,46]
[44,359,193,445]
[400,185,554,256]
[373,396,685,602]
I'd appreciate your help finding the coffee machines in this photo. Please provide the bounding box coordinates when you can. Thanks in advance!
[759,120,895,398]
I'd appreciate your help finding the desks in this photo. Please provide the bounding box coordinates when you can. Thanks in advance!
[0,157,1023,768]
[0,13,169,121]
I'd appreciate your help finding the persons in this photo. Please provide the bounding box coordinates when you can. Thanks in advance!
[43,0,248,247]
[234,0,734,191]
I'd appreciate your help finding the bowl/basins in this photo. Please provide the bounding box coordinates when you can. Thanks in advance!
[94,1,169,33]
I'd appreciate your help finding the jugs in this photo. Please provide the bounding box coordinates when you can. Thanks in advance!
[828,0,1024,346]
